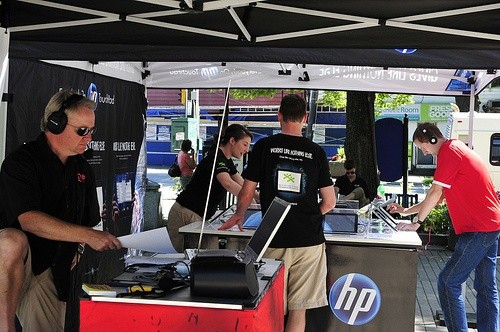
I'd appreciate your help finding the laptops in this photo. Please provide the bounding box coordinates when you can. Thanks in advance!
[236,196,292,266]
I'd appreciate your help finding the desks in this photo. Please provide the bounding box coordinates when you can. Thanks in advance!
[178,210,422,332]
[80,253,285,332]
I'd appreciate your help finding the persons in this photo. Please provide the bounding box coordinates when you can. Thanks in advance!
[333,160,383,209]
[0,90,122,332]
[178,139,197,190]
[217,94,336,332]
[168,124,260,253]
[387,122,500,332]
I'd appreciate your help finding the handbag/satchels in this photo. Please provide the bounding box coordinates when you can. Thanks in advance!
[168,155,182,177]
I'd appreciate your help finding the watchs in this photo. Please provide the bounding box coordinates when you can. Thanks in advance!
[413,215,423,225]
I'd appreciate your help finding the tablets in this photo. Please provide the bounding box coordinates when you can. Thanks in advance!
[241,208,262,228]
[322,213,358,234]
[374,206,399,232]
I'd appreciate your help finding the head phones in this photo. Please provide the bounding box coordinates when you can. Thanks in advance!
[419,124,438,144]
[46,94,84,135]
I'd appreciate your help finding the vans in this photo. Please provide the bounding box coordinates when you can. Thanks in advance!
[441,109,500,196]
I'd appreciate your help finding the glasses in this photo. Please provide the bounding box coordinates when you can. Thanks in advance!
[67,123,97,136]
[346,171,355,174]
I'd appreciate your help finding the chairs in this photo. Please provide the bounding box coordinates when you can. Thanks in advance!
[386,193,418,225]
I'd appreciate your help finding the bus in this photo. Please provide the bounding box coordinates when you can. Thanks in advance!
[145,103,348,171]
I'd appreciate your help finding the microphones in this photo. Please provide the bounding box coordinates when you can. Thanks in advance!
[83,144,93,160]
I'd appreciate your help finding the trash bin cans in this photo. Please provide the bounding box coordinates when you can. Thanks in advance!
[143,179,163,229]
